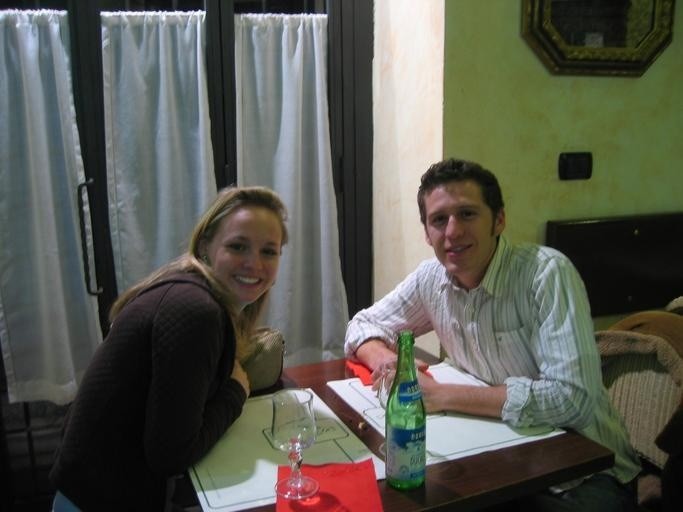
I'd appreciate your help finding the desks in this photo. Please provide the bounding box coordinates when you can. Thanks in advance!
[182,341,616,512]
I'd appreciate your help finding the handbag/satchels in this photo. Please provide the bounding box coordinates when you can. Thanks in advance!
[241,327,285,393]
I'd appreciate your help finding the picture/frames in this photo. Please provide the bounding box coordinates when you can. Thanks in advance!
[519,0,674,74]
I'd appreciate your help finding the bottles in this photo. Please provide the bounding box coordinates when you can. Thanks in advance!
[385,331,425,488]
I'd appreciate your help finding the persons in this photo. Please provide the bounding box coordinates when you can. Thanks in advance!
[339,150,646,511]
[44,179,296,512]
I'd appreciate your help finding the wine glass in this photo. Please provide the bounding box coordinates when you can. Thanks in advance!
[379,361,418,456]
[271,389,319,498]
